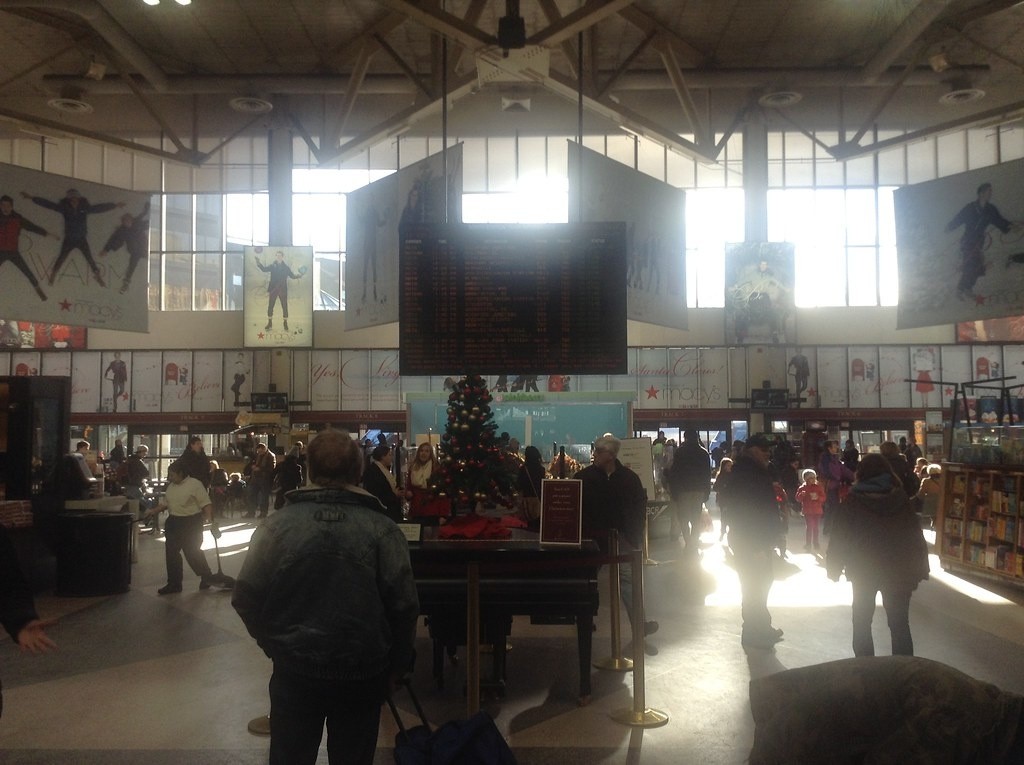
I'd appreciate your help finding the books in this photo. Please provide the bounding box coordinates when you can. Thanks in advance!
[943,471,1024,578]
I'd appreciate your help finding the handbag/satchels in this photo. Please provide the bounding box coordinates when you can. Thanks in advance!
[700,502,714,533]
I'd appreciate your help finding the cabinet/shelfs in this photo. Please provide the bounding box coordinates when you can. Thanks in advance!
[936,463,1024,588]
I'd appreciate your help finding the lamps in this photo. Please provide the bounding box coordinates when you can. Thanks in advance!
[230,88,275,113]
[939,77,986,106]
[48,86,93,113]
[759,84,801,107]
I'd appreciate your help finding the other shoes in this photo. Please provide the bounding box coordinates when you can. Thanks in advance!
[199,573,212,589]
[242,512,255,518]
[158,581,182,594]
[258,512,267,518]
[643,621,658,636]
[742,624,782,638]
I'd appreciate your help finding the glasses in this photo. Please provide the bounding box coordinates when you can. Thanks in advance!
[684,429,697,437]
[596,449,610,455]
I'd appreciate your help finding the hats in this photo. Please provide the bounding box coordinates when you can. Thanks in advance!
[805,471,816,481]
[747,433,779,447]
[134,445,149,453]
[927,464,942,474]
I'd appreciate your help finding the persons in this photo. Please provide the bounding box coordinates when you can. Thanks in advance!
[148,429,443,765]
[627,222,663,293]
[401,189,423,223]
[0,186,152,301]
[105,352,128,412]
[574,436,658,637]
[729,262,790,344]
[787,345,811,399]
[351,191,388,302]
[110,439,155,527]
[255,251,305,331]
[230,353,250,409]
[943,183,1012,301]
[76,441,90,458]
[507,437,546,528]
[0,523,57,716]
[652,425,942,658]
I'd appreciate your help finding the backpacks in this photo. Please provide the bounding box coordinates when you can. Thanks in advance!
[116,455,140,485]
